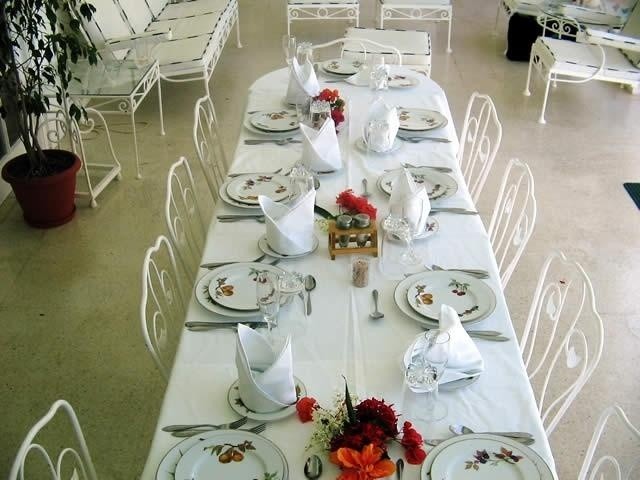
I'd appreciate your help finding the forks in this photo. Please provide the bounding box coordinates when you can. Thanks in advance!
[171,422,268,439]
[163,414,249,432]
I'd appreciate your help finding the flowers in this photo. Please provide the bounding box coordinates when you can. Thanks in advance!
[291,387,429,480]
[309,87,348,135]
[315,186,380,236]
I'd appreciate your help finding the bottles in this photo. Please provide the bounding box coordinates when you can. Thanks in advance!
[168,27,172,41]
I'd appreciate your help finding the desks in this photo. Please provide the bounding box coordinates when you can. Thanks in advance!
[136,60,561,480]
[30,56,169,181]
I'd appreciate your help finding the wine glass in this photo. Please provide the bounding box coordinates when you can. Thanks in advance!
[422,327,452,421]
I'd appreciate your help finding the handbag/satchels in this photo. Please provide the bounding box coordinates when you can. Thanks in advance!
[506,12,587,63]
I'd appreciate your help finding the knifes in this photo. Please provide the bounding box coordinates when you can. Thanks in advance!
[182,321,278,328]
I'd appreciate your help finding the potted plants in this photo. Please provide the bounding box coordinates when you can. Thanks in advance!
[0,0,103,228]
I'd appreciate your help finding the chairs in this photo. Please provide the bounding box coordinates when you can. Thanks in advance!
[162,154,207,286]
[495,1,639,126]
[484,155,540,292]
[457,88,504,210]
[190,92,230,207]
[519,247,605,442]
[573,399,639,479]
[7,396,103,479]
[66,0,219,137]
[136,233,190,382]
[300,36,403,67]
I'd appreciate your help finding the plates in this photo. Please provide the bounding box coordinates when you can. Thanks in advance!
[421,434,554,480]
[154,429,288,479]
[403,339,480,392]
[228,374,308,423]
[194,59,498,328]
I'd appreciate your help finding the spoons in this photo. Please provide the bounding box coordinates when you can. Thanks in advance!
[305,454,322,480]
[448,424,534,437]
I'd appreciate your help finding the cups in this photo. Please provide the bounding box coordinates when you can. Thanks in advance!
[406,350,423,392]
[279,35,314,67]
